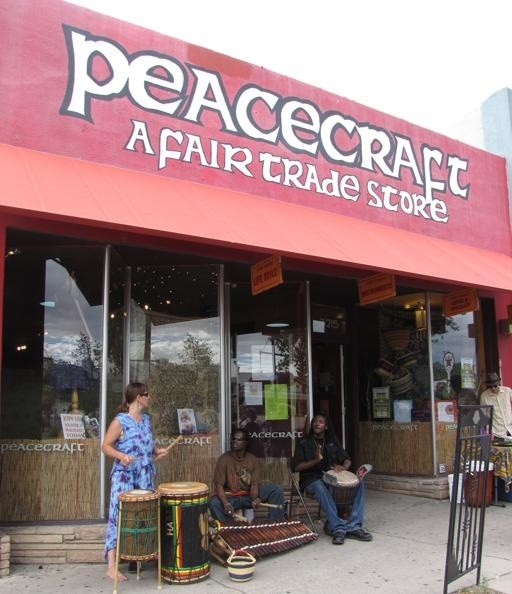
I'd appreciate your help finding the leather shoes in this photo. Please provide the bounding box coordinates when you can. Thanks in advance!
[332,527,346,545]
[347,529,373,541]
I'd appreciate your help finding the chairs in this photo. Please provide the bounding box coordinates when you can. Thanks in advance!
[285,458,324,536]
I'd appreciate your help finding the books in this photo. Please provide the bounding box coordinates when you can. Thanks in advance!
[176,408,198,434]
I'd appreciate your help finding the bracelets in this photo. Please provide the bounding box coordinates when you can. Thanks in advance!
[224,502,231,507]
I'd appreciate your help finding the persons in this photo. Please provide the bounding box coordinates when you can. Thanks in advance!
[242,406,266,458]
[291,412,371,544]
[209,427,288,524]
[181,412,195,434]
[99,382,169,582]
[477,372,512,441]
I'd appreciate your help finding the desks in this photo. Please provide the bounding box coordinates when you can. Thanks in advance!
[463,440,512,511]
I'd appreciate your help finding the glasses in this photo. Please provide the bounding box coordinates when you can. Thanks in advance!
[232,437,249,441]
[488,384,498,388]
[139,392,150,398]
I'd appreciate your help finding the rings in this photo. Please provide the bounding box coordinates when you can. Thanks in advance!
[227,509,231,514]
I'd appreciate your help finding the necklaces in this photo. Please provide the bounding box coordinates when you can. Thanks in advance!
[311,435,325,452]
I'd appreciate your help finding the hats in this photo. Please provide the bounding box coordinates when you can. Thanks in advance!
[485,372,501,383]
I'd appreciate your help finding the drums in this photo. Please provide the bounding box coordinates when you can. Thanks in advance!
[118,489,159,560]
[159,481,209,584]
[323,470,360,509]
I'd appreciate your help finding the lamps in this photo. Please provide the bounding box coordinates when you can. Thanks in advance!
[413,300,427,331]
